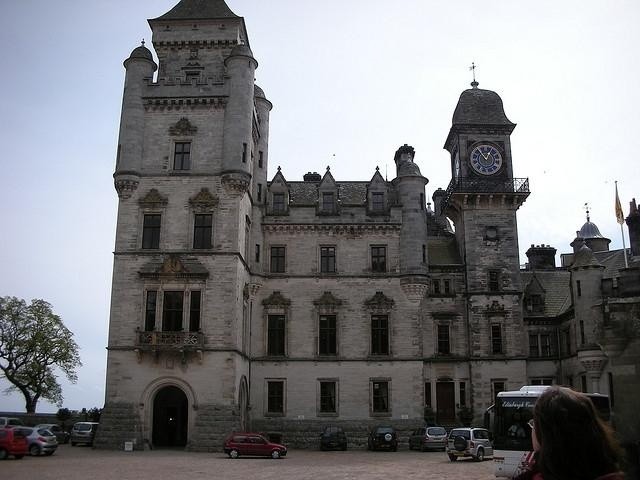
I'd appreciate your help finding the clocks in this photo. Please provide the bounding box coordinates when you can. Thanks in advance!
[467,142,505,177]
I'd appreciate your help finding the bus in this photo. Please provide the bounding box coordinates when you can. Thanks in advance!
[493,386,611,477]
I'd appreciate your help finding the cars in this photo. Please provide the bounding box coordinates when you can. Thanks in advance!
[223,432,287,459]
[320,426,347,451]
[0,417,99,459]
[368,427,397,452]
[447,427,493,462]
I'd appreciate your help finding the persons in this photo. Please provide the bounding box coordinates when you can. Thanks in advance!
[514,385,628,480]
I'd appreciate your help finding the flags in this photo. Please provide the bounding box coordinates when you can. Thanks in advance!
[613,184,626,225]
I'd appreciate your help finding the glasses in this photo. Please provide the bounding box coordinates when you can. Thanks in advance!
[527,420,535,430]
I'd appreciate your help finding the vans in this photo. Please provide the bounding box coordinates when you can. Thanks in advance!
[409,427,447,452]
